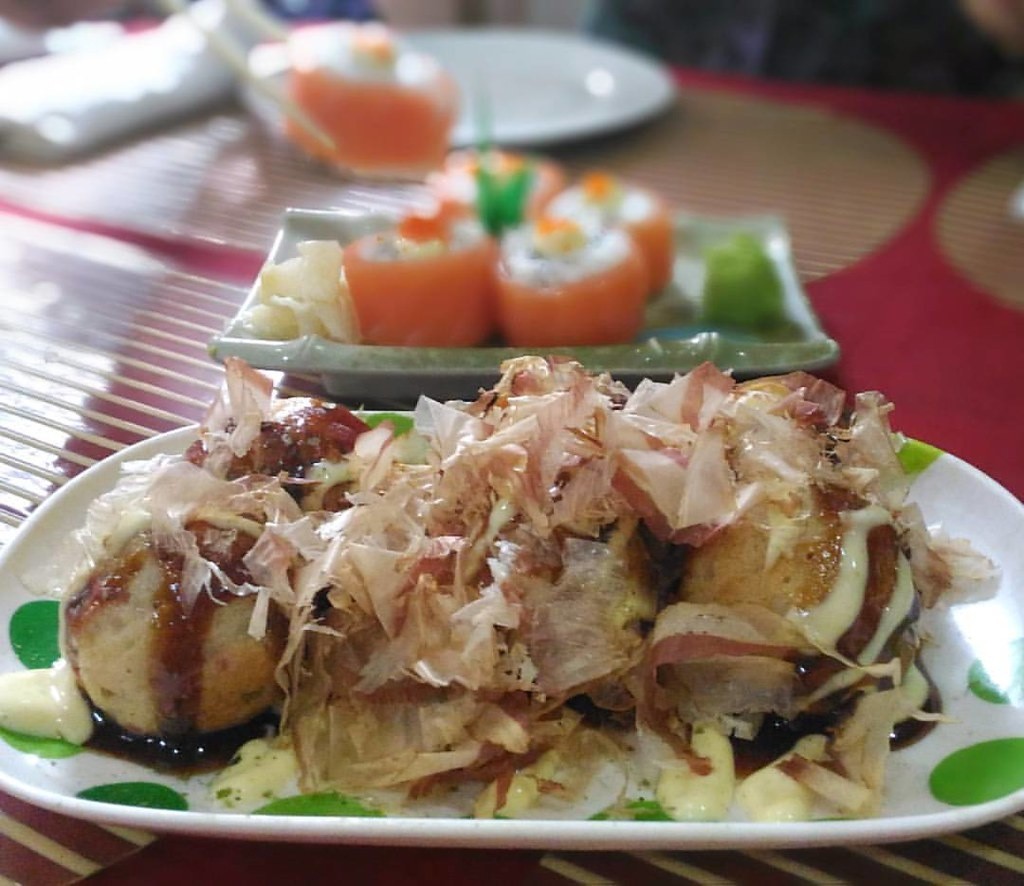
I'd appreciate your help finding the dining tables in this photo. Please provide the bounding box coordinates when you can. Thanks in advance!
[1,59,1024,886]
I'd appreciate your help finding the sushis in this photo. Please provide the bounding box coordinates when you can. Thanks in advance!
[287,27,455,169]
[544,177,674,302]
[491,220,647,349]
[344,204,497,349]
[439,149,561,222]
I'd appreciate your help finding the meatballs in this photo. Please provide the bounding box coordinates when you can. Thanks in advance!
[62,500,286,735]
[175,391,376,517]
[668,463,919,696]
[720,371,859,463]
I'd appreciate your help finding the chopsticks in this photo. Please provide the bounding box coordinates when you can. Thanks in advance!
[164,3,340,150]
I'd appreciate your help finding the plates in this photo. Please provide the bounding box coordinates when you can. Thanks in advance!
[1,427,1023,848]
[210,206,842,397]
[396,27,684,158]
